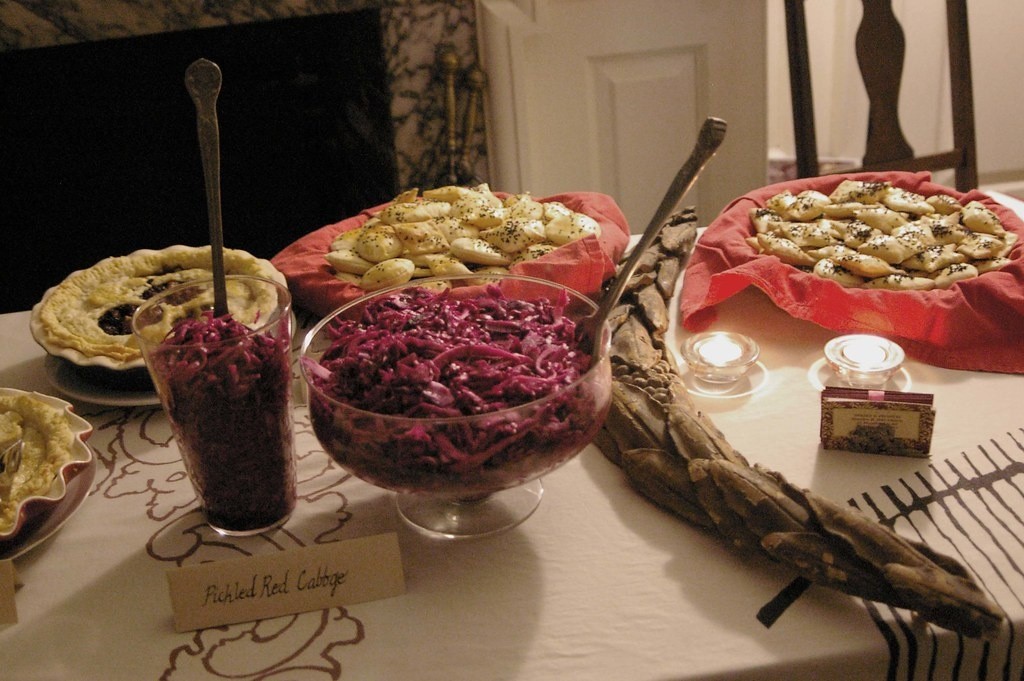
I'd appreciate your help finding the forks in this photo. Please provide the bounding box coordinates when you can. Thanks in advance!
[576,117,727,376]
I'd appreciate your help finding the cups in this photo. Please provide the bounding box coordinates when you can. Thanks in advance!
[131,275,297,537]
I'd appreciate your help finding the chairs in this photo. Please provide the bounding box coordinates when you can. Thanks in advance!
[785,0,980,193]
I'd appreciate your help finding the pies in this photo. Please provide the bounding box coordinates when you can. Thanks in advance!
[39,247,274,360]
[0,396,70,532]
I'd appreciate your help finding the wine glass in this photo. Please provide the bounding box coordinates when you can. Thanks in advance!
[301,276,612,539]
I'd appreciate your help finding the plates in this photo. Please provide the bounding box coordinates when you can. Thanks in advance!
[0,442,98,561]
[31,245,288,371]
[0,387,93,537]
[45,310,296,405]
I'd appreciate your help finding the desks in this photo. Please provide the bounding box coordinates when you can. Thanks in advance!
[0,188,1024,681]
